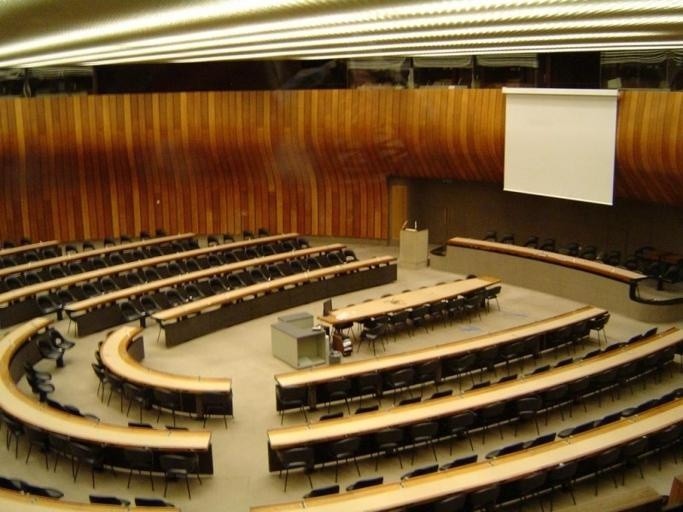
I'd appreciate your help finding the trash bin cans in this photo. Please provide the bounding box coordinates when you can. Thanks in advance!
[329,351,342,364]
